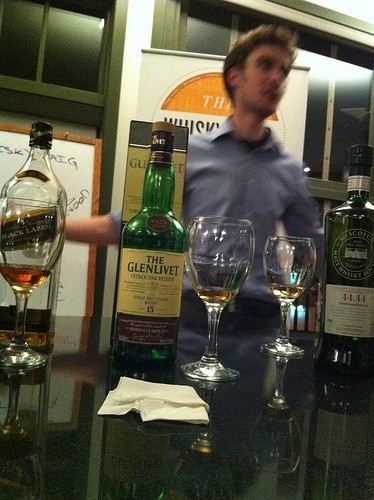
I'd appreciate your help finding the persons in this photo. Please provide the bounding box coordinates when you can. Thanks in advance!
[56,28,324,332]
[51,333,321,500]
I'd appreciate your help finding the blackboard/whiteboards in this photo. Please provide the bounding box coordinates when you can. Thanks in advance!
[0,121,104,316]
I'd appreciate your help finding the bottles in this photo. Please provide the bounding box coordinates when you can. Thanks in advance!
[304,382,374,500]
[0,121,68,353]
[111,121,186,366]
[314,144,374,380]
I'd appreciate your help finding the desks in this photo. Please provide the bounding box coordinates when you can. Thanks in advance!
[0,315,374,500]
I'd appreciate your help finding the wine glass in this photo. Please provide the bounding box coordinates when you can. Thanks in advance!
[0,196,64,372]
[0,366,43,500]
[248,355,301,474]
[260,234,316,359]
[155,379,235,500]
[179,217,254,382]
